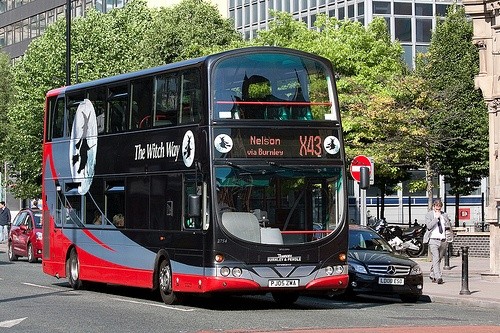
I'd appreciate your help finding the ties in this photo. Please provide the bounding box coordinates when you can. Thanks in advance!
[438,218,443,234]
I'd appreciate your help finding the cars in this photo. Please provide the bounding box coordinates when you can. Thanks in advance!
[7,207,44,264]
[313,222,423,303]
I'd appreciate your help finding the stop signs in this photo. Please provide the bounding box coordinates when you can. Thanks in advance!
[350,155,372,182]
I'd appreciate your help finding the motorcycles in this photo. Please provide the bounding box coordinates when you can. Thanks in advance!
[368,216,427,258]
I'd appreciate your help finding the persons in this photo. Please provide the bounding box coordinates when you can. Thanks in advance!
[0,201,11,244]
[92,210,103,225]
[217,187,230,214]
[32,202,38,209]
[112,214,125,226]
[422,200,452,285]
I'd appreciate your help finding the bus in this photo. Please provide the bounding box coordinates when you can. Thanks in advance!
[41,45,370,305]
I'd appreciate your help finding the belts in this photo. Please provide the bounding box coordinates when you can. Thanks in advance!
[429,238,446,242]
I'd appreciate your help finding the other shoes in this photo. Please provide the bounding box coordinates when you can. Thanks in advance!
[430,276,436,282]
[438,278,443,284]
